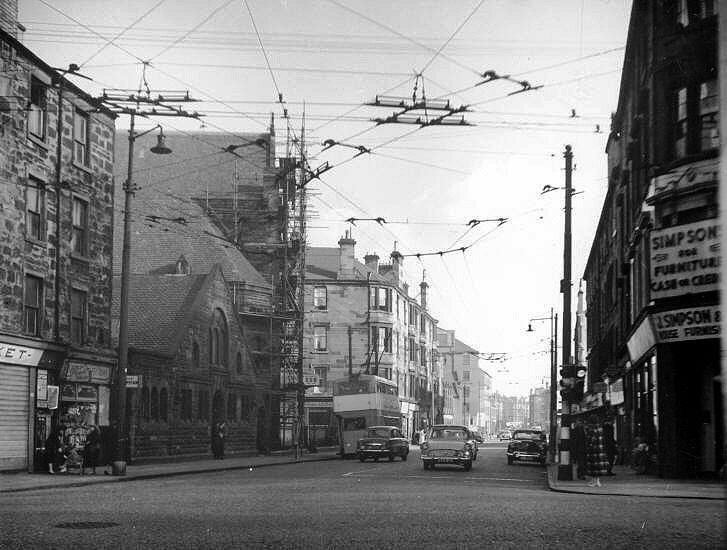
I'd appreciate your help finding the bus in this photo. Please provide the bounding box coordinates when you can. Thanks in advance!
[333,374,402,454]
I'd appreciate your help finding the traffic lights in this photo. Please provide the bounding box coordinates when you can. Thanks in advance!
[560,367,574,398]
[574,367,587,402]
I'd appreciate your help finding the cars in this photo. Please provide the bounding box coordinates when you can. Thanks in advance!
[420,425,477,470]
[500,431,512,441]
[507,429,547,465]
[356,425,409,462]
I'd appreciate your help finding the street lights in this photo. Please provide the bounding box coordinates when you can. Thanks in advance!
[113,115,172,475]
[526,308,556,464]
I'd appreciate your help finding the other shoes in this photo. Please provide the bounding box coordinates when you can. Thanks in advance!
[586,478,604,488]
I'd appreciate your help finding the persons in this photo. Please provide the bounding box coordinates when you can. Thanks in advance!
[212,421,225,458]
[46,431,62,474]
[586,415,609,487]
[571,418,587,478]
[85,424,102,474]
[606,416,619,476]
